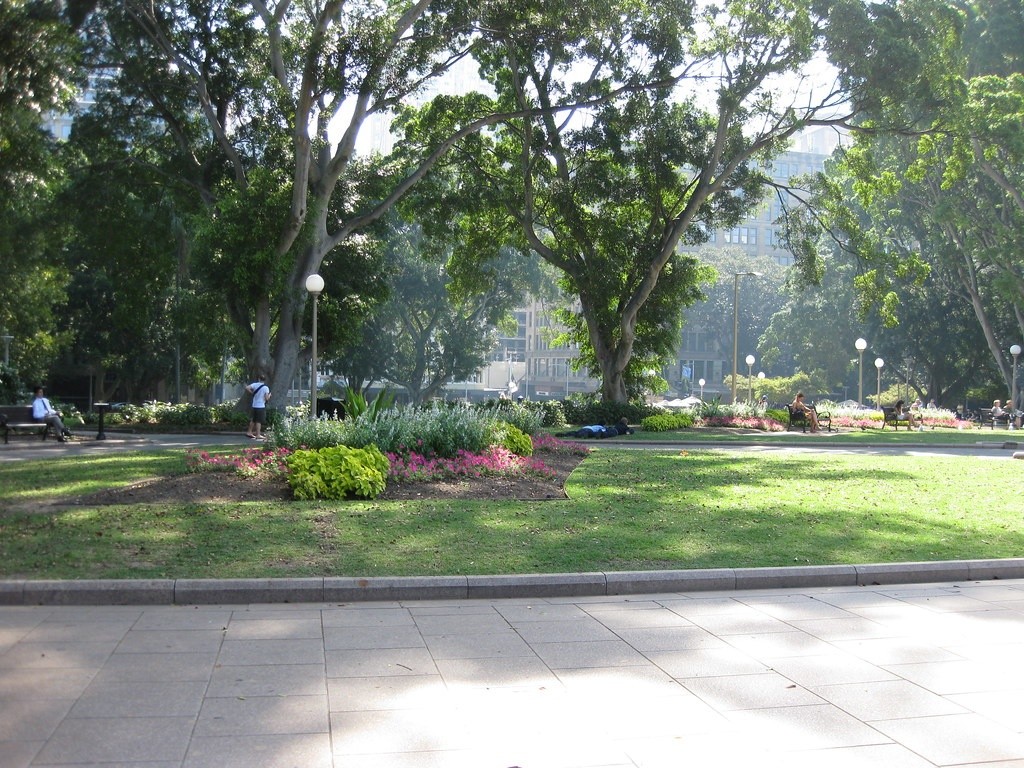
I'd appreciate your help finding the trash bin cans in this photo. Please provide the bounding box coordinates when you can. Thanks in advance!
[317,397,345,421]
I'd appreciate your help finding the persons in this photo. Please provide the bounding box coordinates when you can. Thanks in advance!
[793,393,822,433]
[245,375,272,439]
[584,417,634,439]
[895,400,919,431]
[956,401,982,422]
[1003,400,1023,427]
[759,395,768,409]
[33,386,75,442]
[927,397,937,411]
[555,419,606,438]
[991,400,1016,430]
[911,399,921,409]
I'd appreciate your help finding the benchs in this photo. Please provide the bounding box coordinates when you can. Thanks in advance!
[881,406,924,431]
[0,406,63,443]
[979,408,1017,430]
[786,404,831,433]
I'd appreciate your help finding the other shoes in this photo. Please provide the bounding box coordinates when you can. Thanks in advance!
[246,434,255,438]
[58,436,66,441]
[65,432,73,435]
[256,436,265,439]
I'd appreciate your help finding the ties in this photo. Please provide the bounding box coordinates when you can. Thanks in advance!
[42,399,48,411]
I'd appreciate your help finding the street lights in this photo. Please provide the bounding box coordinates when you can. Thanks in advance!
[731,271,764,407]
[874,358,884,411]
[649,370,655,407]
[2,328,14,368]
[757,372,766,402]
[746,355,755,407]
[1008,344,1022,415]
[854,338,867,409]
[698,378,705,400]
[306,275,325,420]
[518,395,523,417]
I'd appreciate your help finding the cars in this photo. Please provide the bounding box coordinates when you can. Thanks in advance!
[110,402,128,409]
